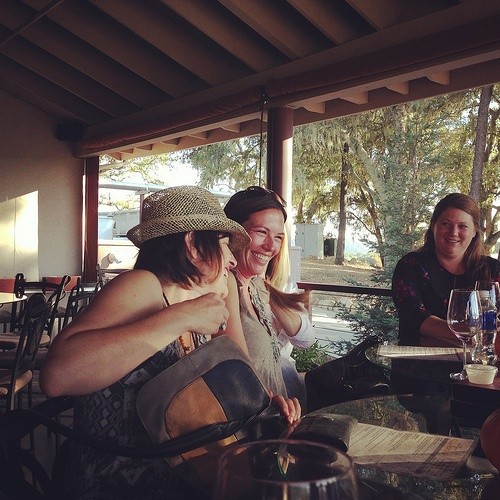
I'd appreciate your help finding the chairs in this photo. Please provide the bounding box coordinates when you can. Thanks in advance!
[0,264,104,500]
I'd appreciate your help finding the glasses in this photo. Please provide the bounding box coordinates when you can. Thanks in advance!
[242,186,286,208]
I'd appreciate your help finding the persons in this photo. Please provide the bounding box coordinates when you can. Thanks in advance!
[40,186,302,500]
[390,193,500,398]
[223,185,390,415]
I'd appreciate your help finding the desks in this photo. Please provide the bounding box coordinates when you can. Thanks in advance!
[364,336,473,414]
[0,292,28,308]
[280,393,500,500]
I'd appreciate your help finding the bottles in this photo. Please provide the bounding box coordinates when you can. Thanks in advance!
[471,290,498,365]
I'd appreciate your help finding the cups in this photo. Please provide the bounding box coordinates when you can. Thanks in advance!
[213,438,361,500]
[474,280,500,333]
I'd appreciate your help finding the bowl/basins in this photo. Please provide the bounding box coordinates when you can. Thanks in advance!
[465,364,499,385]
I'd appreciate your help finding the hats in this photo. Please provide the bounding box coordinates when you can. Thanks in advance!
[481,409,500,470]
[127,185,252,251]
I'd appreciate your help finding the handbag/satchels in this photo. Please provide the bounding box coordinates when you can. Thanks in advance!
[288,412,357,458]
[304,335,392,413]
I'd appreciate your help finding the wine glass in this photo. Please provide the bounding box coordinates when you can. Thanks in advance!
[446,289,480,381]
